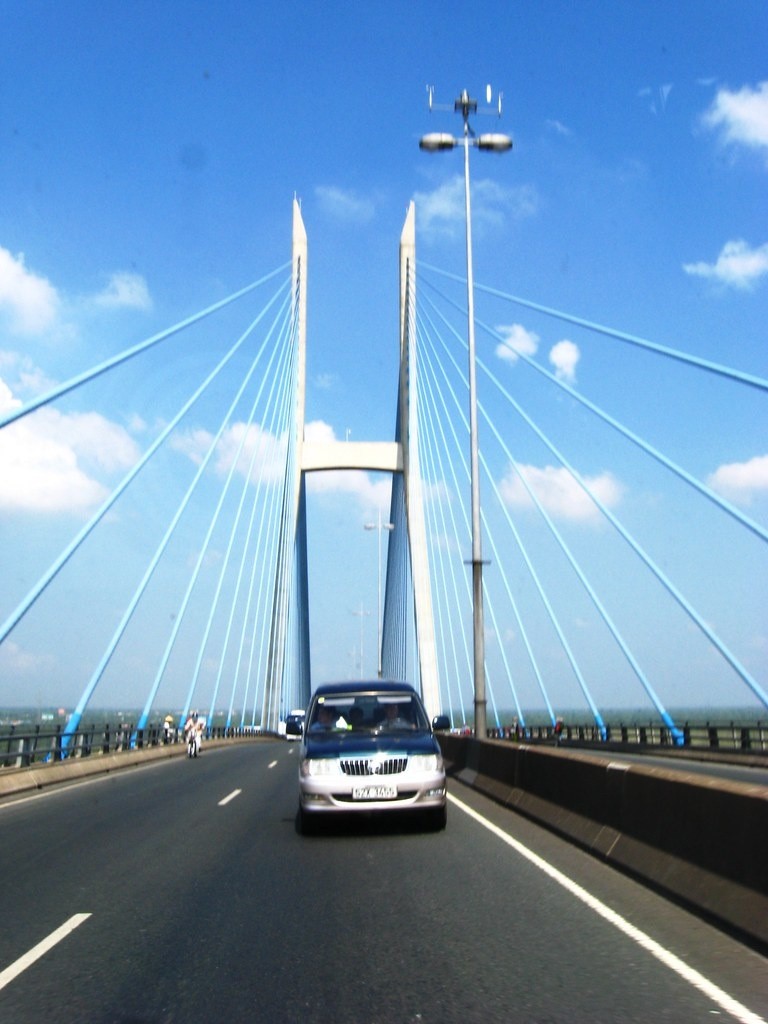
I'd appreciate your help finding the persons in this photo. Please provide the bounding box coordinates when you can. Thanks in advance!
[310,706,337,733]
[554,717,564,748]
[378,704,410,729]
[464,719,470,737]
[184,712,206,753]
[512,717,519,743]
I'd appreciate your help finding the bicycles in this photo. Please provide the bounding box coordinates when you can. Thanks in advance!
[187,729,202,759]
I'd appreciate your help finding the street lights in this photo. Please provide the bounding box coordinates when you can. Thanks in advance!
[364,504,394,679]
[352,606,370,680]
[419,85,513,736]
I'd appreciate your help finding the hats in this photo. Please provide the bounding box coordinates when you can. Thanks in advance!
[192,712,198,718]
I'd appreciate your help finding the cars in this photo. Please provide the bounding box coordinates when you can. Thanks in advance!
[286,681,451,829]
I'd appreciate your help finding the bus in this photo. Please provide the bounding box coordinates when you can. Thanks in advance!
[284,710,306,741]
